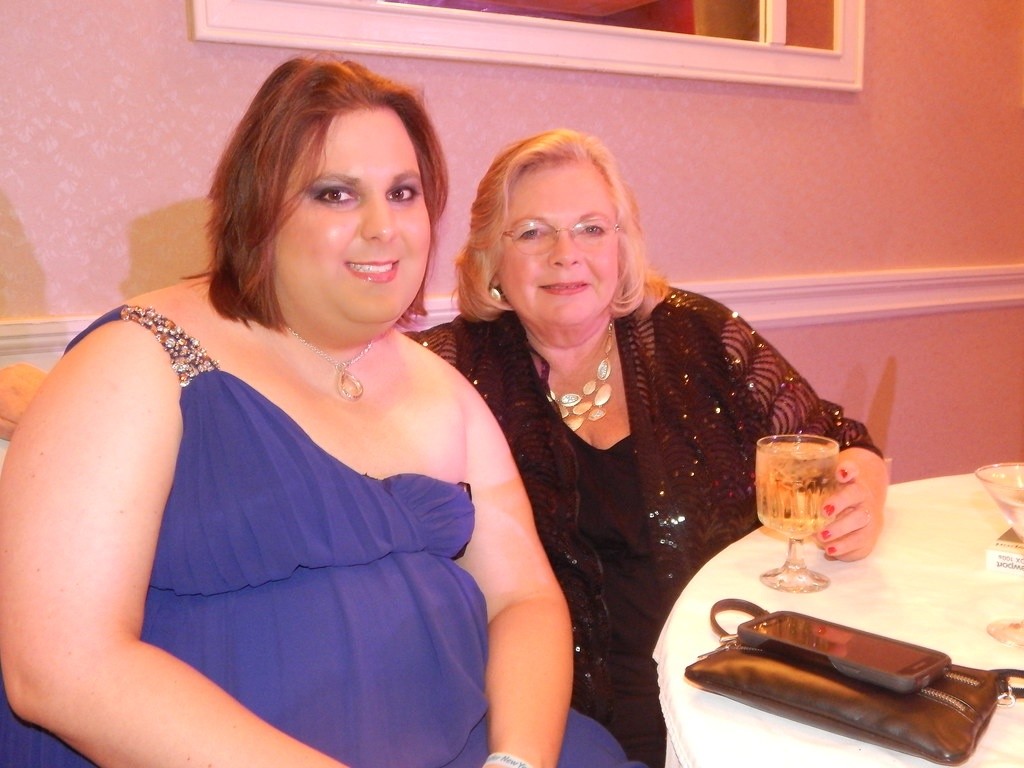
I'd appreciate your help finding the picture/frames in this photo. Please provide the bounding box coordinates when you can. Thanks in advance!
[191,0,866,94]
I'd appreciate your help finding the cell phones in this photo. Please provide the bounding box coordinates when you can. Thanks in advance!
[737,610,952,694]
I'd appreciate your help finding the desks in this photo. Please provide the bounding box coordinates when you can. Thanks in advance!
[651,469,1024,767]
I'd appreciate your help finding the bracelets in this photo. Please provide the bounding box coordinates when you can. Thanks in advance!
[482,752,531,768]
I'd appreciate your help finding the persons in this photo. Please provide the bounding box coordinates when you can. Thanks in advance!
[405,130,888,768]
[0,59,628,768]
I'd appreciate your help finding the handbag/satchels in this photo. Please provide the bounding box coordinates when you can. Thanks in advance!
[681,597,1024,768]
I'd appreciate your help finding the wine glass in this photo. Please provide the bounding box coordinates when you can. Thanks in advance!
[974,462,1024,649]
[755,435,839,593]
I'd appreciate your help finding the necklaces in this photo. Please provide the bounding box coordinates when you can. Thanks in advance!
[546,321,613,431]
[287,324,372,401]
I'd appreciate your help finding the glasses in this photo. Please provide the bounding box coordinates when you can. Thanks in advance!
[500,216,622,255]
